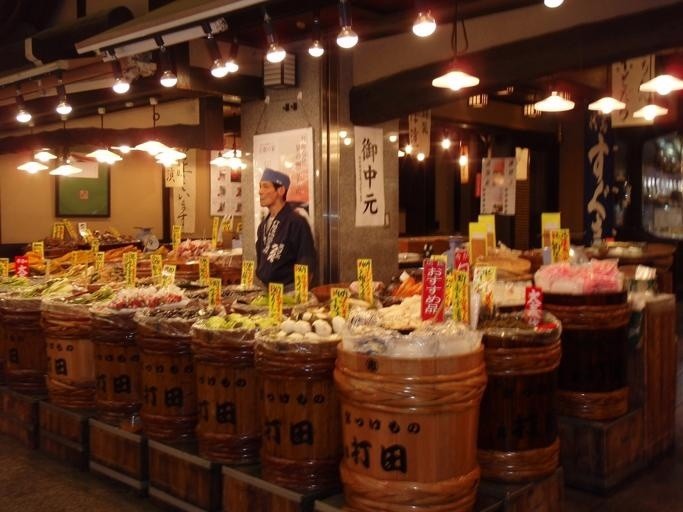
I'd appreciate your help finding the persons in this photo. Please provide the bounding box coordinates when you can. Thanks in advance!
[652,193,665,209]
[255,169,317,293]
[654,190,682,237]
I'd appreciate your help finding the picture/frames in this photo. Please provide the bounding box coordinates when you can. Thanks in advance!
[50,145,112,221]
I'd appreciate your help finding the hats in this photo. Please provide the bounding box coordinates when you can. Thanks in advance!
[261,167,290,188]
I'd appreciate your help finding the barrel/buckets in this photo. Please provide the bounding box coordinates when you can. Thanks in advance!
[0,254,634,512]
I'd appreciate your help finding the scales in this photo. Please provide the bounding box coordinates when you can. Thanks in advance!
[133,225,160,252]
